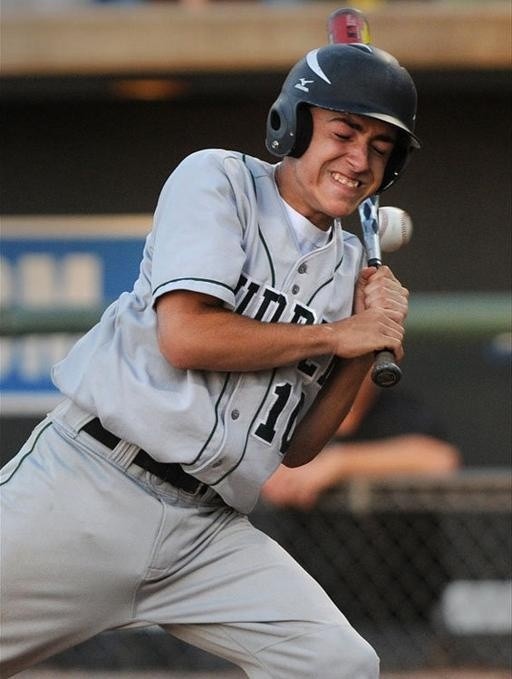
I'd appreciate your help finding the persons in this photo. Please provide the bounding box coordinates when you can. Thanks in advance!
[0,43,424,679]
[260,378,461,616]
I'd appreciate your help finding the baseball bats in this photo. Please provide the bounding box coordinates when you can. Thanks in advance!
[327,8,402,388]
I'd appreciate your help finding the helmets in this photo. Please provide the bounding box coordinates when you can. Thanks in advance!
[264,42,423,196]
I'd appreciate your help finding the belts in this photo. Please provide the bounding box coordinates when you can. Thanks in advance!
[82,416,221,505]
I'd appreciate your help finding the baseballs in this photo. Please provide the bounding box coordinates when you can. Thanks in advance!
[377,206,412,252]
